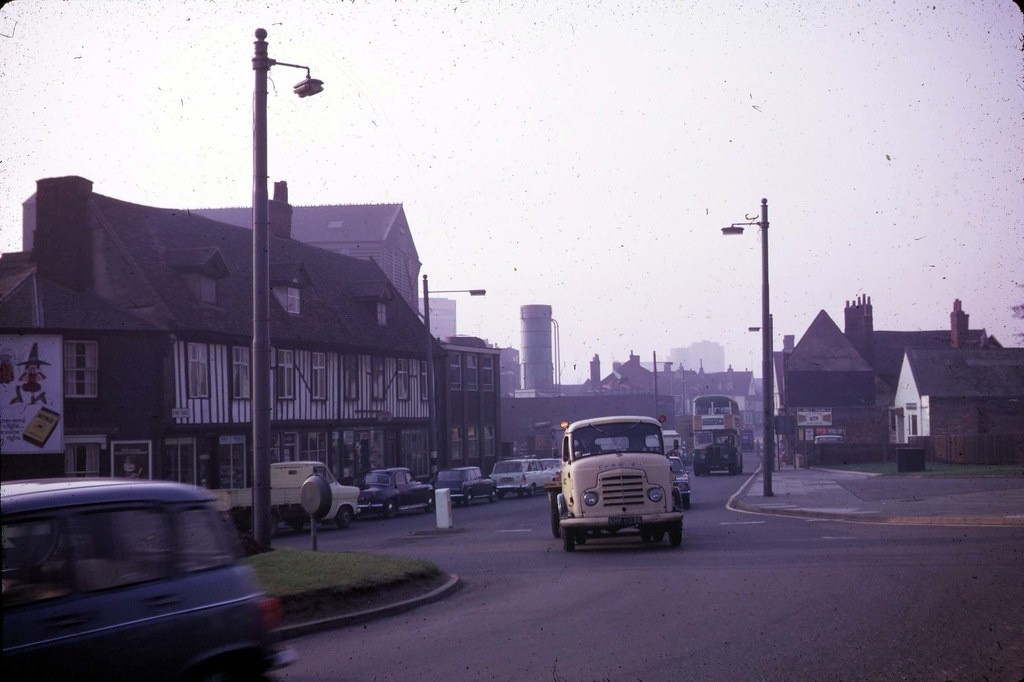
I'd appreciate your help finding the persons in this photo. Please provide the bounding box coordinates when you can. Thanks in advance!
[573,434,603,458]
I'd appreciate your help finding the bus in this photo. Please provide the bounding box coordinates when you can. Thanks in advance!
[689,395,744,477]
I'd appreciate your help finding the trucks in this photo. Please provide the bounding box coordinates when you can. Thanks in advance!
[208,461,360,539]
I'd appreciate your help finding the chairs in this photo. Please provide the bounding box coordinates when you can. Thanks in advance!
[626,435,650,452]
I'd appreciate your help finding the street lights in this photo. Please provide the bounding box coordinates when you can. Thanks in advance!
[424,289,487,485]
[252,57,325,550]
[747,326,773,384]
[721,221,774,499]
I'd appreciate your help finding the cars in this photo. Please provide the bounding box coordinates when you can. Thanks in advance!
[668,456,692,510]
[487,457,557,499]
[347,465,435,520]
[542,459,563,490]
[814,436,845,445]
[1,479,297,682]
[741,431,754,451]
[430,466,497,509]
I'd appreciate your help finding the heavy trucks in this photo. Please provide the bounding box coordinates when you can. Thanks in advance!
[546,416,684,551]
